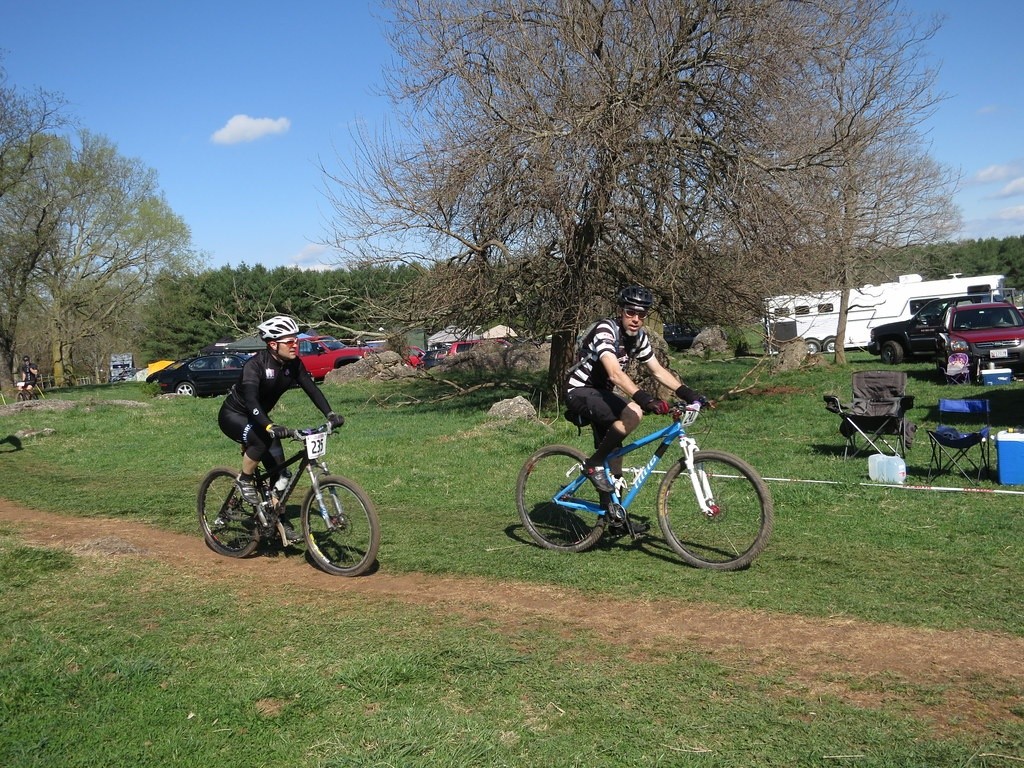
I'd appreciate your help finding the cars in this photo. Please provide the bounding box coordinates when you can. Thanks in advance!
[416,348,449,369]
[662,325,699,351]
[146,354,248,397]
[297,336,386,382]
[404,346,425,369]
[112,368,140,381]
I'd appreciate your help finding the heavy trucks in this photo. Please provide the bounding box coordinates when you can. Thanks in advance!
[764,273,1005,354]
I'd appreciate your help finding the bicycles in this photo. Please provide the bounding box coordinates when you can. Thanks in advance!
[517,401,773,572]
[15,382,39,402]
[196,418,381,577]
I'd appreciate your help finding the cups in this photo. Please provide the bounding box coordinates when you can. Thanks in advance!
[988,362,994,369]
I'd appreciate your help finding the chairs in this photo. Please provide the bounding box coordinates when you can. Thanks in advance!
[940,354,970,384]
[991,312,1003,324]
[824,369,916,460]
[925,398,990,486]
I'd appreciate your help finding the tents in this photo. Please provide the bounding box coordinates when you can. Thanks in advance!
[479,324,518,339]
[428,325,480,346]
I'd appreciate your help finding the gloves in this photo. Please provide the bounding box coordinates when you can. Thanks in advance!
[633,389,668,417]
[675,386,709,410]
[264,422,289,440]
[327,412,345,428]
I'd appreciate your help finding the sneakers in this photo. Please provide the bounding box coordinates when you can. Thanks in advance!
[274,525,299,540]
[235,474,259,503]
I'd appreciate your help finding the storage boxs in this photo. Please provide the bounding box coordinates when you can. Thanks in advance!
[995,429,1024,486]
[980,368,1013,387]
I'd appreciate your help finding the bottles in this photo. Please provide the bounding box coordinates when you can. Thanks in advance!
[272,468,292,499]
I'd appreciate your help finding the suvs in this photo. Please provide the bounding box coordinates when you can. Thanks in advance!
[934,297,1024,385]
[449,339,531,373]
[869,292,1005,365]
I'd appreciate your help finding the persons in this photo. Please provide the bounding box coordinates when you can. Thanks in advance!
[563,287,705,534]
[18,355,38,399]
[218,315,345,543]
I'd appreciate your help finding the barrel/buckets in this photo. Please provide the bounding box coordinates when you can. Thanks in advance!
[868,454,906,483]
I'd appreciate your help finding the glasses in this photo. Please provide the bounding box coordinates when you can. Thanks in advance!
[620,308,648,320]
[267,338,299,347]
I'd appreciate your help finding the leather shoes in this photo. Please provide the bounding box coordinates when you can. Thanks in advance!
[582,458,614,493]
[610,521,648,535]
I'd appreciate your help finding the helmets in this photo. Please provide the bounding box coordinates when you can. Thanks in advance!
[618,286,653,310]
[256,315,299,340]
